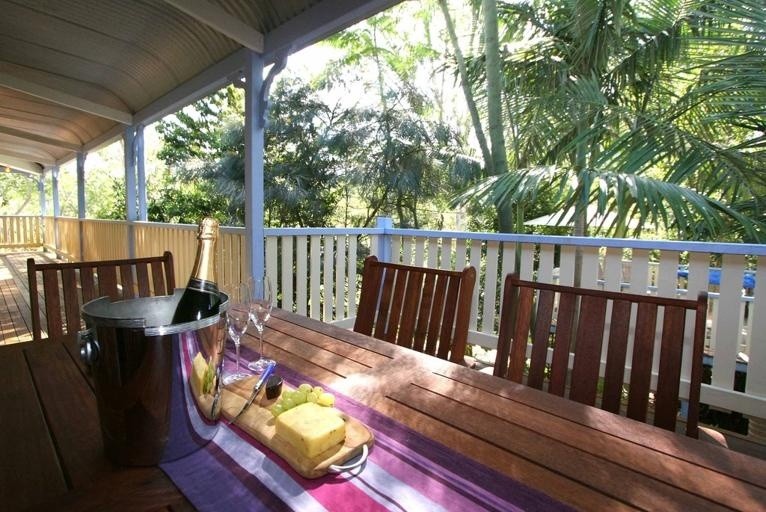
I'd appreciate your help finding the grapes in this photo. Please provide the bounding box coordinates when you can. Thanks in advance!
[271,384,335,417]
[203,365,213,394]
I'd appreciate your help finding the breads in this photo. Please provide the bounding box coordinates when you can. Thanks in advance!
[193,352,208,396]
[275,402,345,458]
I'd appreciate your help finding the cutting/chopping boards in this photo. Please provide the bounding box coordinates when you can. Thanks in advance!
[214,373,374,478]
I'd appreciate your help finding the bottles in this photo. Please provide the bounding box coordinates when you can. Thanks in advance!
[171,217,221,326]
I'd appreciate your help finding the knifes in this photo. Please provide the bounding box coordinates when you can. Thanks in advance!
[227,361,276,425]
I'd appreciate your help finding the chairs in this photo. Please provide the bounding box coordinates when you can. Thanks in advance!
[352,255,475,363]
[26,251,177,342]
[493,275,708,442]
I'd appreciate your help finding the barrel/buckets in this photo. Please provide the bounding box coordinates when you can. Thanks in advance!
[78,291,228,469]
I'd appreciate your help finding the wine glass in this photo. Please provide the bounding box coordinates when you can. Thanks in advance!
[223,277,275,388]
